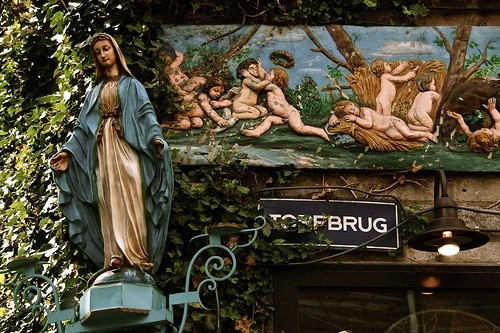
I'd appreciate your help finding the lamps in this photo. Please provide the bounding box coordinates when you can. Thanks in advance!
[403,168,492,258]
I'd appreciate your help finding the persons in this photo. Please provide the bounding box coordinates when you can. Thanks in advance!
[49,32,176,276]
